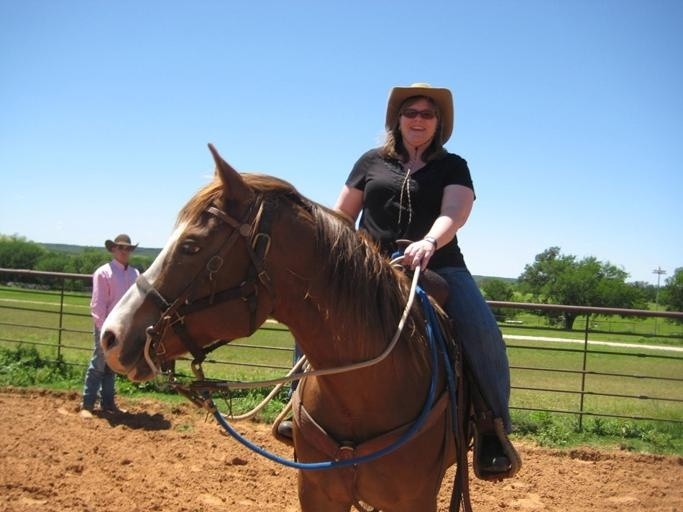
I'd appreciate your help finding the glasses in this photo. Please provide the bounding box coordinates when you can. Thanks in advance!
[400,107,440,120]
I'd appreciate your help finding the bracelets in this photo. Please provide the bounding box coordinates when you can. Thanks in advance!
[420,236,438,251]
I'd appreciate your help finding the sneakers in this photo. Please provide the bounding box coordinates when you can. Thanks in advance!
[477,436,512,472]
[277,414,294,437]
[79,407,128,421]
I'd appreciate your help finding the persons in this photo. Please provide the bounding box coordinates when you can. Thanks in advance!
[79,232,140,422]
[275,79,515,476]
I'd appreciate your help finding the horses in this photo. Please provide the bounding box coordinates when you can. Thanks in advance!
[100,143,475,512]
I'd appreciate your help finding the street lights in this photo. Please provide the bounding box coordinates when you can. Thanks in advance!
[652,266,666,334]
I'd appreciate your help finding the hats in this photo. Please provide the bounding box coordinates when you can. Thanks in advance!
[105,233,140,254]
[381,81,455,148]
